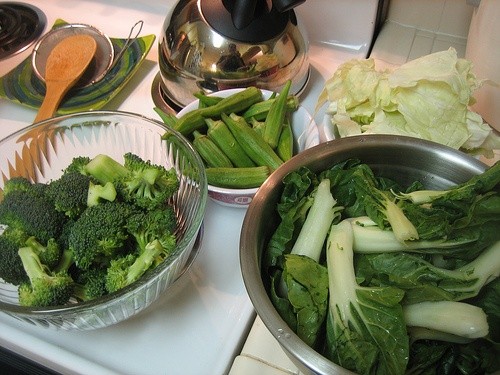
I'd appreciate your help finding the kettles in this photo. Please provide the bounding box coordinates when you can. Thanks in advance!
[157,0,312,109]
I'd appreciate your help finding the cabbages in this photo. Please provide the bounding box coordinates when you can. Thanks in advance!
[316,47,497,160]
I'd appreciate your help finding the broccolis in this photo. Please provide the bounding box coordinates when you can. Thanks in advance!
[0,152,178,307]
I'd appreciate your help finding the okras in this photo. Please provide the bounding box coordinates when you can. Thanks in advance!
[153,79,299,188]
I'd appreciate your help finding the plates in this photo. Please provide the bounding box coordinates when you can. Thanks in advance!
[151,64,319,114]
[176,215,204,292]
[0,18,156,111]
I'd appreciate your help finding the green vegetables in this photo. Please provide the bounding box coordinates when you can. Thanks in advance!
[271,155,499,375]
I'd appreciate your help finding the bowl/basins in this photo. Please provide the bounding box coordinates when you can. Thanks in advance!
[0,110,208,333]
[323,90,480,161]
[239,133,500,375]
[164,88,321,211]
